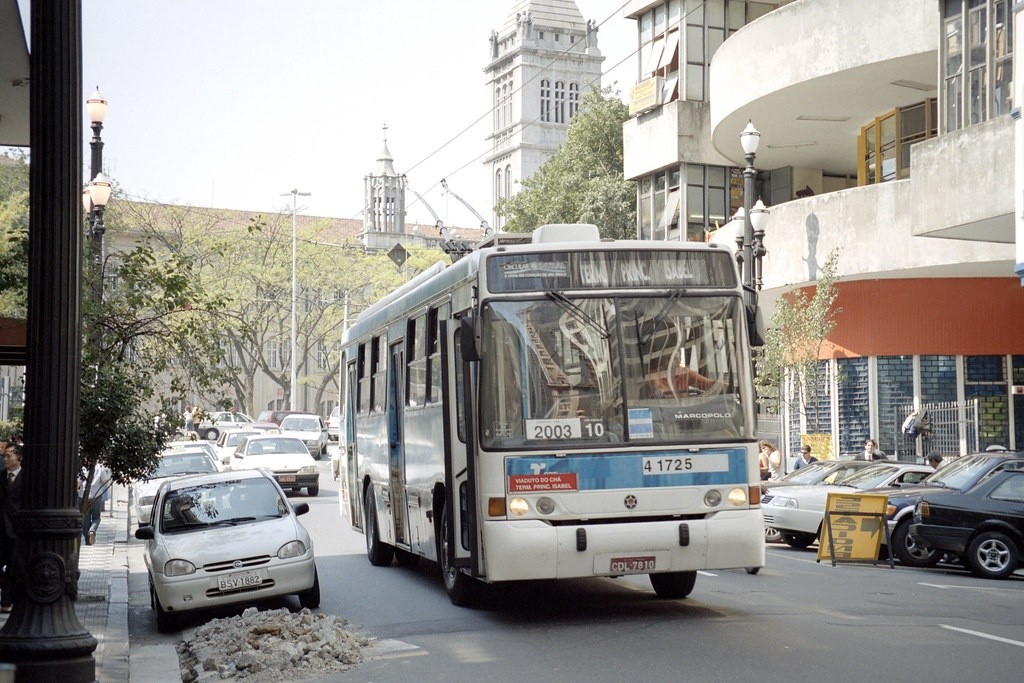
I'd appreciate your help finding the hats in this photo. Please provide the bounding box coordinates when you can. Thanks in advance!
[928,451,943,461]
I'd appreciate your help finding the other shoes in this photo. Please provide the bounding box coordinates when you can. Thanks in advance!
[85,531,96,546]
[1,605,13,613]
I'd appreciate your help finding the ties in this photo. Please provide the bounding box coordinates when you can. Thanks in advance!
[868,455,872,461]
[7,472,15,483]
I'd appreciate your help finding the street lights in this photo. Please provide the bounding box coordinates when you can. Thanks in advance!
[733,115,772,352]
[277,187,315,412]
[83,81,112,354]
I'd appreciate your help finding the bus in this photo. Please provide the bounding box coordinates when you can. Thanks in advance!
[338,219,770,607]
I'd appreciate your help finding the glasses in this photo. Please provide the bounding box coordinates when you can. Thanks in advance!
[801,451,809,454]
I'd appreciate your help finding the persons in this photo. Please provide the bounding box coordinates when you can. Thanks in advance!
[648,345,728,399]
[183,405,203,431]
[760,440,785,483]
[793,445,819,471]
[77,459,108,545]
[0,438,10,472]
[0,443,25,614]
[927,450,960,564]
[853,439,888,461]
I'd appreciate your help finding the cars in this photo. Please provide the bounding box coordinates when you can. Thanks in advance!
[324,406,340,441]
[279,413,328,460]
[212,428,266,464]
[133,439,225,528]
[257,409,314,428]
[853,452,1024,568]
[228,434,320,496]
[133,466,322,633]
[760,459,937,548]
[908,466,1024,579]
[197,410,257,440]
